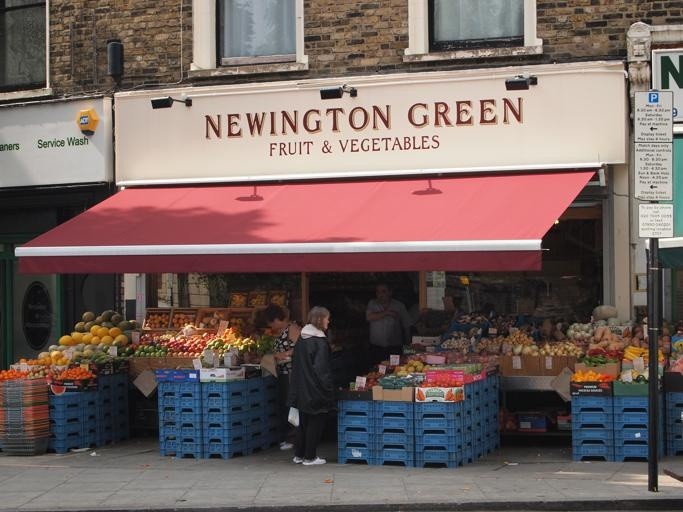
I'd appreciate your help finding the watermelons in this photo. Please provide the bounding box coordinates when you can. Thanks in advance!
[74,310,139,332]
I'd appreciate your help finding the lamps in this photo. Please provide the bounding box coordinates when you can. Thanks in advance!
[504,72,538,90]
[320,84,357,100]
[151,96,192,108]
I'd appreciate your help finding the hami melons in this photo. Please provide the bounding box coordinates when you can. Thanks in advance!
[59,324,128,346]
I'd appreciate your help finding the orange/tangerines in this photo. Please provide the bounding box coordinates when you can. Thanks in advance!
[0,366,96,379]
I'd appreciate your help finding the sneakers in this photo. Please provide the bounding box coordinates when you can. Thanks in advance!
[293,455,302,465]
[279,440,294,451]
[302,456,328,466]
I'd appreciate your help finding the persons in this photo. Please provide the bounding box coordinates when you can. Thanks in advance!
[262,304,300,451]
[365,283,411,364]
[286,306,337,464]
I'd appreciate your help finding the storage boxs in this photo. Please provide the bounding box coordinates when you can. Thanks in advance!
[556,414,571,431]
[10,307,289,391]
[503,411,553,432]
[228,290,290,308]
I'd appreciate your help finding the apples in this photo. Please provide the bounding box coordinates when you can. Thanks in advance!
[103,338,206,358]
